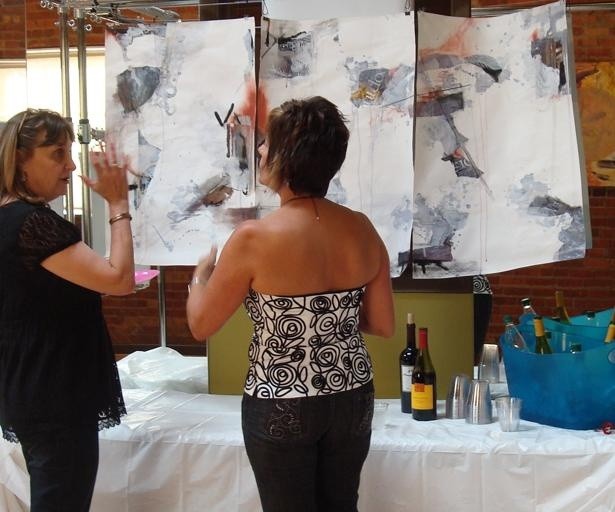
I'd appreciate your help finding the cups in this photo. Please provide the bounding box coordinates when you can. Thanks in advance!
[446,374,469,420]
[496,397,520,433]
[476,344,500,385]
[466,380,492,425]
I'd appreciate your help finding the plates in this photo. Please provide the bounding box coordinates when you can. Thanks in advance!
[134,269,156,285]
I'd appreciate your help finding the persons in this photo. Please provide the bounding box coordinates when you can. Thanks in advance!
[187,97,396,512]
[1,109,135,512]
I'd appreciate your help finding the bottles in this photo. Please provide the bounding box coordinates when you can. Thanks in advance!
[520,297,538,314]
[399,313,418,413]
[412,327,436,420]
[604,316,614,344]
[532,314,551,354]
[503,316,526,350]
[550,291,572,324]
[569,343,584,355]
[584,311,599,328]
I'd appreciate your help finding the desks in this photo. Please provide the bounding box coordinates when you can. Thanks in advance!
[0,347,615,512]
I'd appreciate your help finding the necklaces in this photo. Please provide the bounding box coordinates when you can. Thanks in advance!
[280,195,320,220]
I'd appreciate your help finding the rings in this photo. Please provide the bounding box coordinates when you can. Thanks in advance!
[111,163,122,168]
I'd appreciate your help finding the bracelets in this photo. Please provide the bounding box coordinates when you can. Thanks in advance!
[188,275,208,292]
[108,212,132,224]
